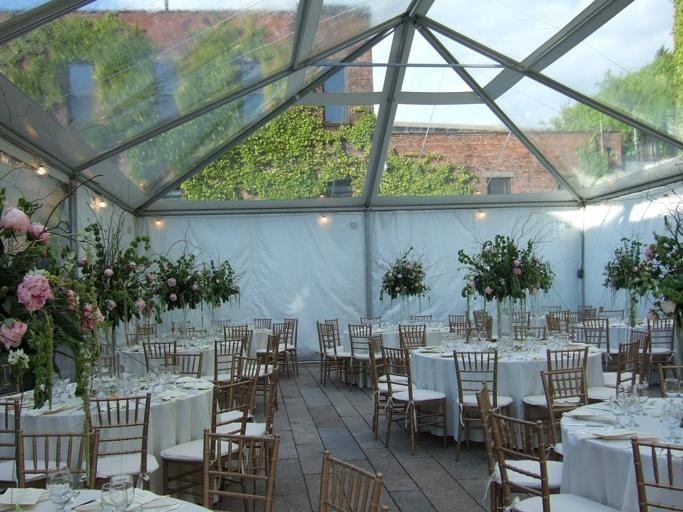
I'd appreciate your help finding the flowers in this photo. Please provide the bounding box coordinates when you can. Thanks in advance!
[632,198,682,323]
[370,246,434,303]
[601,237,650,296]
[455,231,560,304]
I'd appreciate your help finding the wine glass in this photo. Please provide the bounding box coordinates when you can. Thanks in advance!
[612,370,681,446]
[135,323,229,353]
[40,461,138,511]
[48,363,182,408]
[437,329,575,362]
